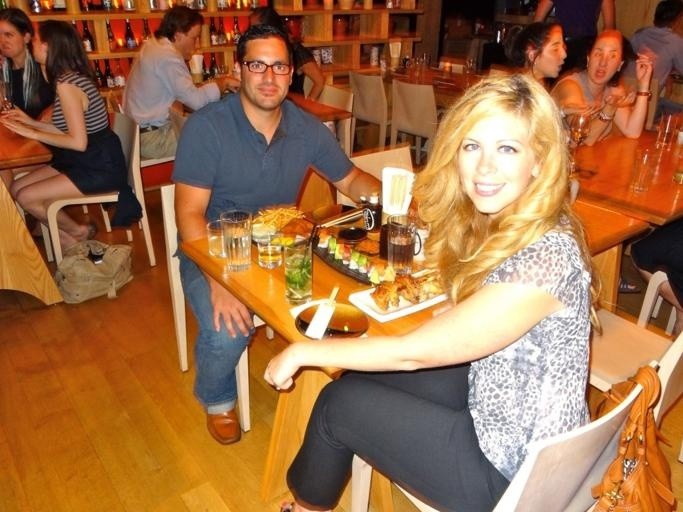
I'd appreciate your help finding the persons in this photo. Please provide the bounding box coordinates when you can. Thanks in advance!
[170,24,428,445]
[0,19,126,241]
[630,215,683,337]
[0,9,55,190]
[505,0,681,292]
[248,7,325,99]
[264,77,590,512]
[121,5,241,159]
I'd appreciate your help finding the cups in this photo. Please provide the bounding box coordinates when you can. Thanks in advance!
[0,96,15,111]
[381,214,428,274]
[207,209,313,305]
[629,113,683,193]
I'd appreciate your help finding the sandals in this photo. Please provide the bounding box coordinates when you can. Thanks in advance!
[618,278,642,294]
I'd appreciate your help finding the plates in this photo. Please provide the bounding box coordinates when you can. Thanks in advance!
[338,227,368,242]
[295,303,370,340]
[348,268,448,323]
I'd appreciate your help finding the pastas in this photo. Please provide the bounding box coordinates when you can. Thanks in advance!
[252,206,305,232]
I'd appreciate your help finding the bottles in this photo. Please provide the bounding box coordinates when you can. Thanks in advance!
[1,0,258,88]
[411,52,428,80]
[363,193,383,232]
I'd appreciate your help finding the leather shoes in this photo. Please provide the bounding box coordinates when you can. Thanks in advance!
[207,401,241,444]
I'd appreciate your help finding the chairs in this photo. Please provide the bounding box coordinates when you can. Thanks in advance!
[569,306,683,435]
[345,364,660,511]
[161,173,275,435]
[138,71,683,262]
[41,114,156,276]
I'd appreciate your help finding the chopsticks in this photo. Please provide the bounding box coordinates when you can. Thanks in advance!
[324,287,340,305]
[316,211,364,228]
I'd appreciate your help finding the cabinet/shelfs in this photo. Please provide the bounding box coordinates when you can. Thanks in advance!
[0,0,496,110]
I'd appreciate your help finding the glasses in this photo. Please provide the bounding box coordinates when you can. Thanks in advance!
[243,58,292,76]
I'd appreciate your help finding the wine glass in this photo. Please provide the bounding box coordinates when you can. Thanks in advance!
[568,114,590,169]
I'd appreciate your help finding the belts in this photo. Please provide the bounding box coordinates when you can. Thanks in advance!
[139,126,158,133]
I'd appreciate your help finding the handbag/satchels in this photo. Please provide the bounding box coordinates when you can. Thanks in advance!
[54,239,132,305]
[591,408,681,512]
